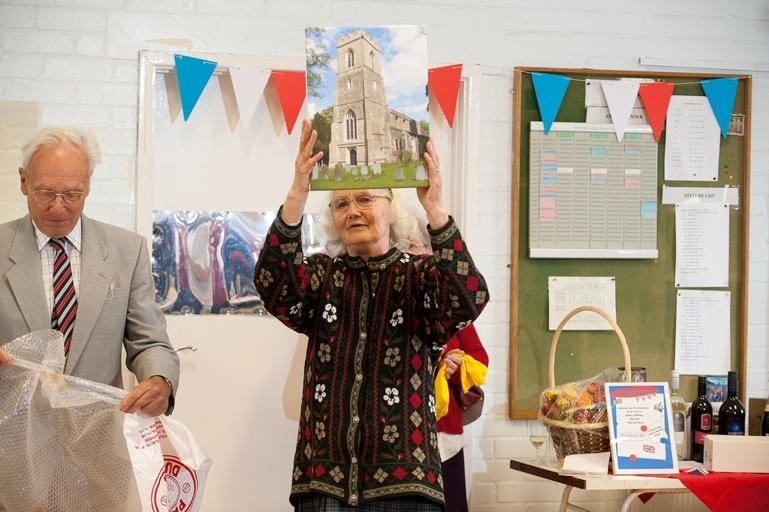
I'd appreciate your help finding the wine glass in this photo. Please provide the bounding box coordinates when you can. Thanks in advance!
[527,417,547,466]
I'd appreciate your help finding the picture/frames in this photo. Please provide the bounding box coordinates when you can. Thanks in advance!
[132,48,474,325]
[604,379,680,476]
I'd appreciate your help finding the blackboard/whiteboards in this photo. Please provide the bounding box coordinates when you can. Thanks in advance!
[511,66,752,420]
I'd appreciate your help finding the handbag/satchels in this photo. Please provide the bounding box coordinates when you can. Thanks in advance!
[456,385,484,426]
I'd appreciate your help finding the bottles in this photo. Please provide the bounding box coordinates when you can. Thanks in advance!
[691,373,713,460]
[719,370,745,436]
[666,369,688,458]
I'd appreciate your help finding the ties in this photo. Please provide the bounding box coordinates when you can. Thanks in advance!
[50,238,78,356]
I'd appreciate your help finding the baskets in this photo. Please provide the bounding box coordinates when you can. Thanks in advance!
[538,306,631,466]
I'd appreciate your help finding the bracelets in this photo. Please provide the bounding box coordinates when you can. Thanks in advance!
[159,376,173,393]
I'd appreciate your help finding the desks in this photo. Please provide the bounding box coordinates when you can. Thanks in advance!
[509,455,768,512]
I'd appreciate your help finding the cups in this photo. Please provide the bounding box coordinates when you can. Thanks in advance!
[620,366,648,383]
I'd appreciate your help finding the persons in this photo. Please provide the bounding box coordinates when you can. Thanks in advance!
[365,199,488,512]
[0,120,182,512]
[255,119,491,512]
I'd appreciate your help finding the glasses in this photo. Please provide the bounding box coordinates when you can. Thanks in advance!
[329,192,391,210]
[28,184,85,204]
